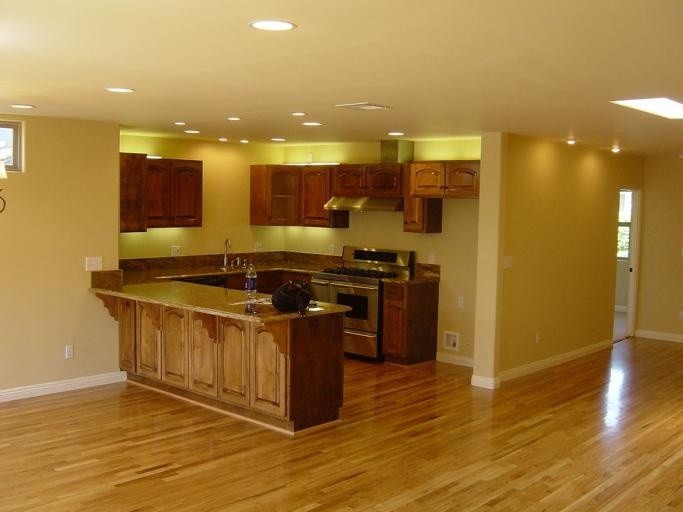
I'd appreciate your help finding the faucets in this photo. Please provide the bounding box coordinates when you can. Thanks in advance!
[223,236,231,265]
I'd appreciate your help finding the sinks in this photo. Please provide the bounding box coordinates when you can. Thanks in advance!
[213,265,238,273]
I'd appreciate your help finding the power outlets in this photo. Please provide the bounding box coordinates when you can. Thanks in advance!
[66,344,74,360]
[457,296,464,309]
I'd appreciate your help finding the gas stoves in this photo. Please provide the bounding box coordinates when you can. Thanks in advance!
[312,245,414,284]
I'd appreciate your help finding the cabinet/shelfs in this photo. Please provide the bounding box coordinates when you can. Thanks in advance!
[249,313,344,432]
[120,152,147,233]
[146,158,203,228]
[409,159,480,200]
[135,300,189,390]
[332,163,402,202]
[118,297,135,382]
[250,165,301,226]
[300,165,349,229]
[381,282,439,365]
[281,271,311,291]
[403,162,442,233]
[188,310,249,408]
[225,271,282,295]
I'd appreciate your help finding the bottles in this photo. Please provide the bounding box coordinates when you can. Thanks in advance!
[245,302,258,315]
[245,260,257,302]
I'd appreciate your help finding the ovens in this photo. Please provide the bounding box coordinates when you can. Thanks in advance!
[311,278,379,361]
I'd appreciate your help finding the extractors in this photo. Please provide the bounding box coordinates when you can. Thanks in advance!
[322,140,414,211]
